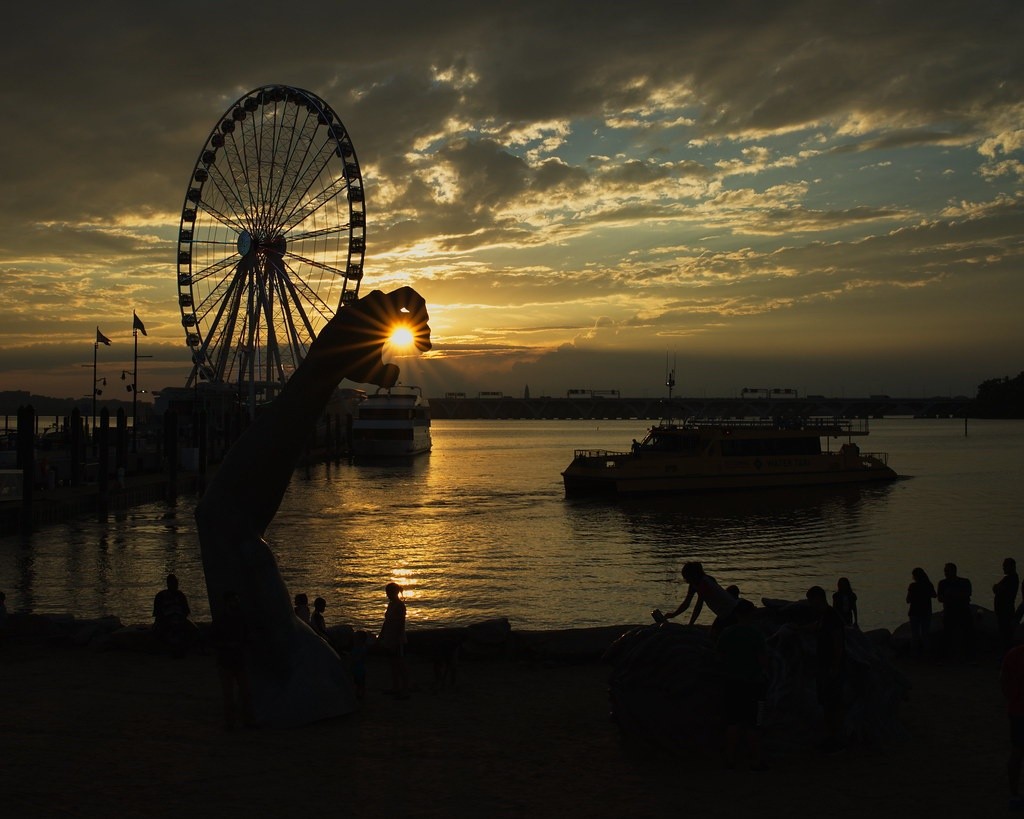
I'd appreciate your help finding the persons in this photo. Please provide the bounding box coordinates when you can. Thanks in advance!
[295,593,310,621]
[379,583,411,695]
[713,600,768,770]
[832,578,859,627]
[999,643,1024,811]
[906,568,936,658]
[311,597,327,634]
[993,558,1024,660]
[937,563,971,629]
[195,286,427,726]
[726,585,740,599]
[209,592,260,732]
[797,586,849,750]
[432,637,458,684]
[666,561,738,639]
[152,574,200,637]
[349,630,369,698]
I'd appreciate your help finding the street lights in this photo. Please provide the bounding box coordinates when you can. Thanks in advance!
[79,342,107,437]
[120,330,151,454]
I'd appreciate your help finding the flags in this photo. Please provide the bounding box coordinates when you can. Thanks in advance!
[133,314,147,335]
[97,330,112,346]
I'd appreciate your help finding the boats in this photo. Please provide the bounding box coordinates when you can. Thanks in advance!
[561,405,899,510]
[349,380,434,458]
